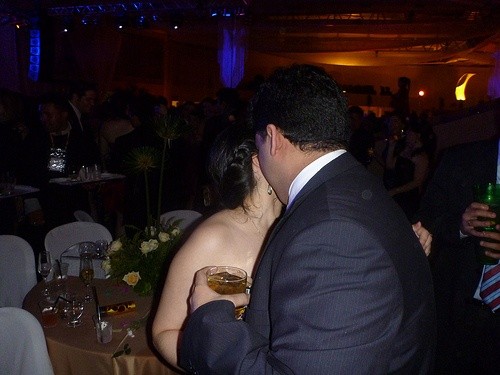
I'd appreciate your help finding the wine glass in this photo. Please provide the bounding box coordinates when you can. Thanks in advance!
[38,251,52,287]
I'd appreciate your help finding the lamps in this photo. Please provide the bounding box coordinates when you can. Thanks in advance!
[62,0,251,34]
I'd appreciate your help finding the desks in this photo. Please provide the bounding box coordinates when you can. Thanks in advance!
[49,172,127,221]
[0,184,41,234]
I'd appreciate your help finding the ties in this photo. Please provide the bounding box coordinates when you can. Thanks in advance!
[479,263,500,311]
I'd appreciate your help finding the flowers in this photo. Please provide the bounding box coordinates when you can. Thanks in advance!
[101,105,190,294]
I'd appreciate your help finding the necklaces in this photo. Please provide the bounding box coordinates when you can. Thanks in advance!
[249,216,265,239]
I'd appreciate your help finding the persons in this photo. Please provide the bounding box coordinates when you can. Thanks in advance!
[0,62,500,375]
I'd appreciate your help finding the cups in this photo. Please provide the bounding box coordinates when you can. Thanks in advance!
[39,287,86,327]
[206,266,248,321]
[53,263,69,288]
[475,182,500,232]
[96,320,113,344]
[78,239,109,288]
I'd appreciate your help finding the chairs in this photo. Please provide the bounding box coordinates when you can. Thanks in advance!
[44,221,113,277]
[0,306,54,375]
[160,210,202,235]
[0,234,38,307]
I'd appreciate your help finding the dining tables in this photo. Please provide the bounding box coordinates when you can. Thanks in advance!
[20,275,177,375]
